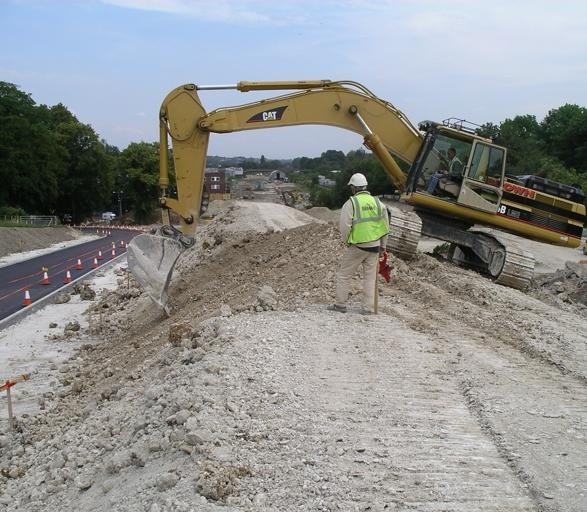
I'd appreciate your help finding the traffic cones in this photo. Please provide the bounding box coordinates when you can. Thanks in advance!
[23,288,33,307]
[96,223,145,260]
[65,269,72,283]
[76,255,83,270]
[41,266,50,285]
[92,256,98,270]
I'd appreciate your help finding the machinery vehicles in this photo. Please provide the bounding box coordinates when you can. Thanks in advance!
[126,74,587,316]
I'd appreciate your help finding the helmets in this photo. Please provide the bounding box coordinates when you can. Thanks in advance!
[347,173,368,187]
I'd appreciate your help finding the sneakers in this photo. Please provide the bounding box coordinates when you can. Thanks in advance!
[361,309,371,315]
[327,304,347,313]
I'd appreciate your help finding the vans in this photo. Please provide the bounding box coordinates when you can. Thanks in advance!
[101,213,116,220]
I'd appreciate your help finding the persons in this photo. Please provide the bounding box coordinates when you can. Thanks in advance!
[327,171,389,314]
[422,147,462,195]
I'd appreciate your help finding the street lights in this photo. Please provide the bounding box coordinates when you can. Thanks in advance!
[112,190,124,217]
